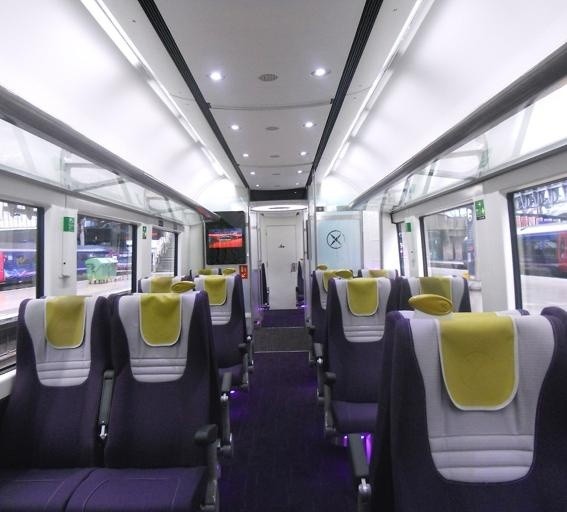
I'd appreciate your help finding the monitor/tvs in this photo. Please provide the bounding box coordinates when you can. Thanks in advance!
[205,225,244,251]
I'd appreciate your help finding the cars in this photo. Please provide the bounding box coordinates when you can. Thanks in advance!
[116,253,132,270]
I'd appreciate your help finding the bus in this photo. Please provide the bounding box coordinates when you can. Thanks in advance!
[517,221,567,273]
[0,248,37,286]
[77,245,114,276]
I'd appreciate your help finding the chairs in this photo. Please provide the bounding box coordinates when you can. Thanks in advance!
[307,266,470,443]
[98,270,254,455]
[294,260,304,308]
[68,294,221,512]
[347,303,567,510]
[1,296,107,512]
